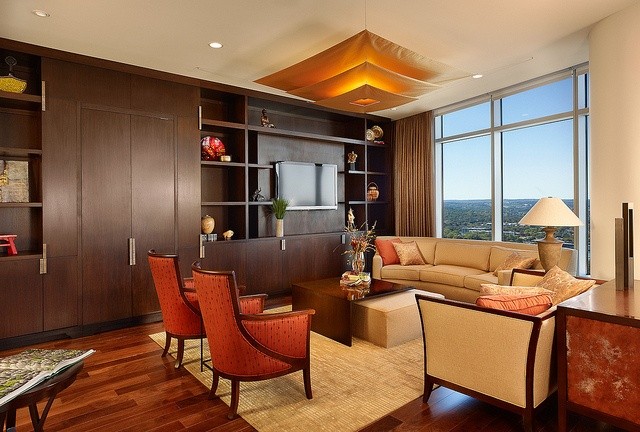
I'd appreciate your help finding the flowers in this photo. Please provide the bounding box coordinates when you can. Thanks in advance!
[338,217,380,256]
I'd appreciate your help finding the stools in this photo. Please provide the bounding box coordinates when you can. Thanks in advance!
[353,289,445,349]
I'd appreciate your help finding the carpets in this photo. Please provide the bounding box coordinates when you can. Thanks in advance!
[148,304,440,432]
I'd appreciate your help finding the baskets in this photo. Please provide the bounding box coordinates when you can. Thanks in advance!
[0,75,28,94]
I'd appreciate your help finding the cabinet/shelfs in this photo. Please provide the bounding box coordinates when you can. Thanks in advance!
[0,37,42,353]
[41,44,201,343]
[201,78,395,297]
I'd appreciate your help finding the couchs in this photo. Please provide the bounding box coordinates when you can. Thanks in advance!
[191,259,315,420]
[146,248,269,369]
[371,236,579,304]
[414,268,608,431]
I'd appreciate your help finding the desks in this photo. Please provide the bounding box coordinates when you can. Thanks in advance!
[0,359,84,432]
[557,277,640,431]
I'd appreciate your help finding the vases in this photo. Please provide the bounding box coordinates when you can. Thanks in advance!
[352,252,365,273]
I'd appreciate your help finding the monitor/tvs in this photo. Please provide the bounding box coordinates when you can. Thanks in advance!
[275,162,339,212]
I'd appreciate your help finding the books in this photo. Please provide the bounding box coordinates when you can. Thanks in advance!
[0,347,96,407]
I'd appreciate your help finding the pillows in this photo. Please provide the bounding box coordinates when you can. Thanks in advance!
[532,265,596,305]
[392,241,426,266]
[475,295,553,316]
[492,252,537,277]
[374,237,402,265]
[479,284,557,295]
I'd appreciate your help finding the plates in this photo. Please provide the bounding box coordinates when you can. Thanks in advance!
[370,125,384,140]
[367,128,375,141]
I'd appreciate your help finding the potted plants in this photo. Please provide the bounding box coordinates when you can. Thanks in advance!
[272,196,290,237]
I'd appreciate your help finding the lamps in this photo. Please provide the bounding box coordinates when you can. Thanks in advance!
[518,197,584,271]
[252,29,474,113]
